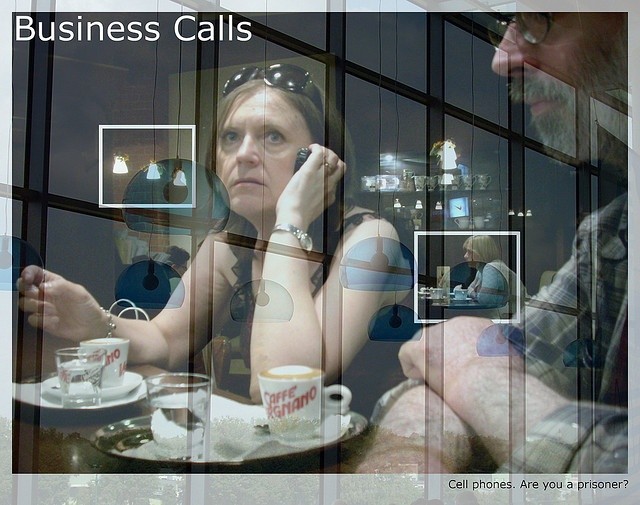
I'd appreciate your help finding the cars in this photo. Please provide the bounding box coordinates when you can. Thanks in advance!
[55,347,107,408]
[410,175,427,191]
[476,173,491,190]
[147,373,212,460]
[257,365,352,444]
[78,338,130,388]
[454,290,469,301]
[425,174,441,191]
[430,288,444,299]
[453,216,470,231]
[402,169,415,193]
[471,217,486,230]
[462,174,477,190]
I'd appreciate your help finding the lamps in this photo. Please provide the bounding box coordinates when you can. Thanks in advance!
[538,270,557,288]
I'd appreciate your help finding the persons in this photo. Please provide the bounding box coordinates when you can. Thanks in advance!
[463,235,527,321]
[15,63,414,419]
[356,12,628,474]
[163,246,190,277]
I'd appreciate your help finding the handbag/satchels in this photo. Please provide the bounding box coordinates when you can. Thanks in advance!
[100,306,116,338]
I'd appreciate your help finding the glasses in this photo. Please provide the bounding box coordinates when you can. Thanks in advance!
[393,198,402,207]
[1,237,41,291]
[509,209,514,215]
[122,0,230,237]
[518,211,524,216]
[367,13,422,341]
[450,12,508,309]
[438,140,457,170]
[115,12,185,309]
[113,153,129,174]
[435,201,442,210]
[338,1,414,293]
[563,334,607,369]
[229,1,295,324]
[526,209,532,216]
[414,200,423,209]
[476,13,525,357]
[172,169,187,188]
[146,161,160,180]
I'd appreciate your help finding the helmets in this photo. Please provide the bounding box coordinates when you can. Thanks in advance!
[13,379,146,410]
[447,292,464,296]
[427,297,448,304]
[42,371,143,400]
[88,411,369,466]
[419,292,430,297]
[452,298,473,303]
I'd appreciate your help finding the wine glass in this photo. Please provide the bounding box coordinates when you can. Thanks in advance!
[324,161,336,170]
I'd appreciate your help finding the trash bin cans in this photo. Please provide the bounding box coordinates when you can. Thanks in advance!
[272,223,313,256]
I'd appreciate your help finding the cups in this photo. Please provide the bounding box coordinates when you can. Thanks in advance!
[223,62,323,114]
[487,11,554,46]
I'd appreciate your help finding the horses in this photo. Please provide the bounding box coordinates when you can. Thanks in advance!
[294,147,313,174]
